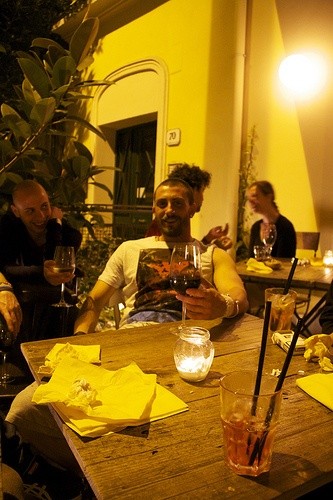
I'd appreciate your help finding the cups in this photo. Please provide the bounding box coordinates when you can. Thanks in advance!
[265,287,297,338]
[261,223,277,247]
[217,369,284,478]
[254,245,272,262]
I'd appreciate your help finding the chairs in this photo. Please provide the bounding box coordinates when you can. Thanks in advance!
[0,261,78,410]
[289,231,321,320]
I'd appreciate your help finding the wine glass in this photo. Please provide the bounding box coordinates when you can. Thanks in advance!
[0,320,17,384]
[169,244,203,336]
[51,246,75,308]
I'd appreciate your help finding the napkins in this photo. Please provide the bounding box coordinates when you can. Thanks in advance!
[296,373,332,411]
[244,257,273,274]
[40,340,189,437]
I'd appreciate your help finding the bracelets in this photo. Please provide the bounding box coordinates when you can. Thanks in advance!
[0,286,15,293]
[73,332,86,336]
[0,281,13,288]
[219,293,235,318]
[225,294,239,318]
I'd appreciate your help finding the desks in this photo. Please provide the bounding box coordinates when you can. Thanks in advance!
[234,256,333,314]
[20,312,333,500]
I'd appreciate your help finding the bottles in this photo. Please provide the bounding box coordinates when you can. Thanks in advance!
[322,250,333,267]
[173,326,214,384]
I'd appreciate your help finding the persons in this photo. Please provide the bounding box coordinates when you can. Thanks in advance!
[248,181,296,259]
[4,177,250,480]
[0,179,83,338]
[144,162,233,252]
